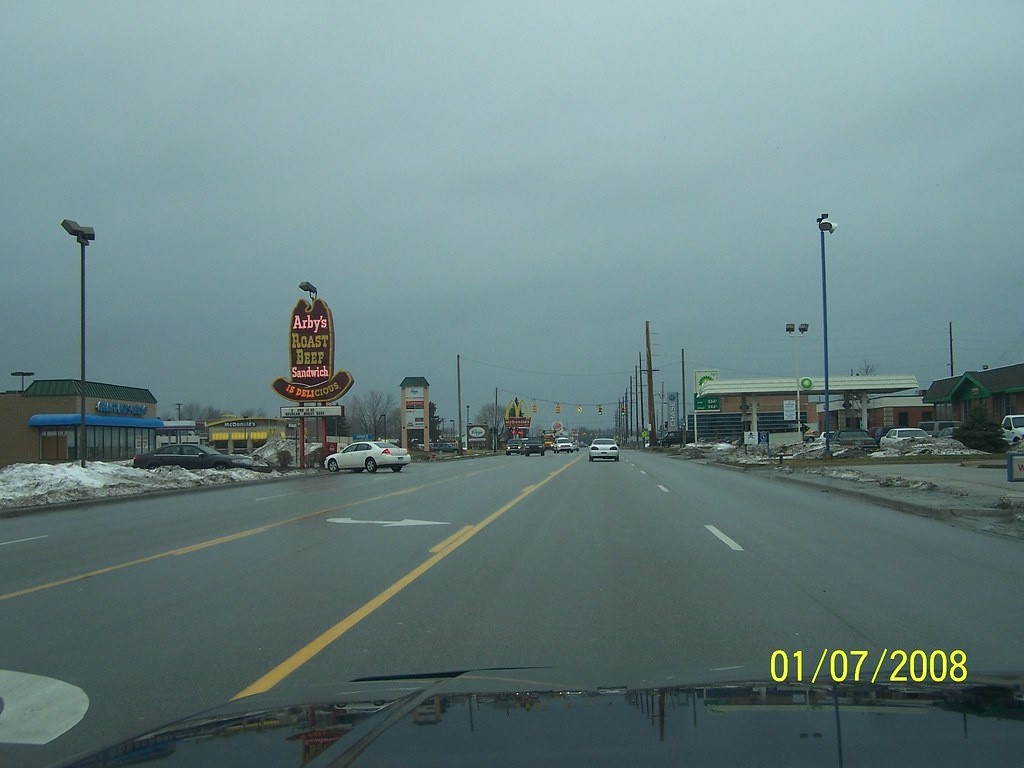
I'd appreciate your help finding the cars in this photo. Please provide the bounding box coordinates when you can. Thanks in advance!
[132,443,254,471]
[998,414,1024,444]
[587,438,620,462]
[523,437,546,456]
[322,440,412,474]
[553,437,579,453]
[818,418,966,450]
[431,443,459,454]
[505,439,525,456]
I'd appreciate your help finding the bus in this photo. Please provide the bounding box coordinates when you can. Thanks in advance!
[542,429,556,448]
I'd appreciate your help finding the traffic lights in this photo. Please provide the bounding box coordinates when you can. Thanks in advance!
[621,407,625,416]
[599,407,602,415]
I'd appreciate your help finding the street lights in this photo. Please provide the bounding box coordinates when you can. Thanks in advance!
[450,419,454,441]
[381,414,386,442]
[816,213,840,461]
[61,219,95,469]
[786,322,810,445]
